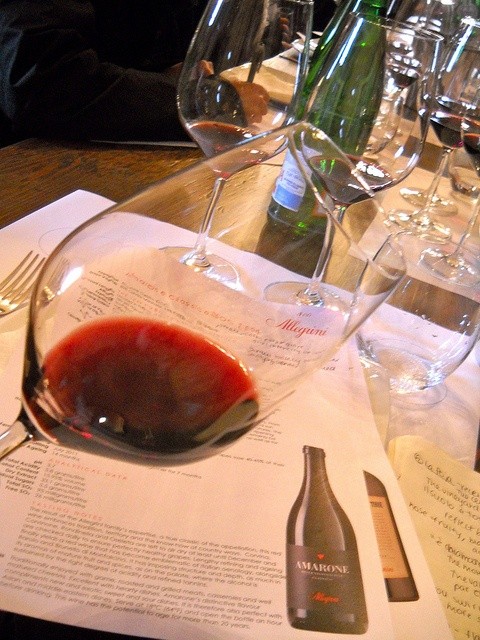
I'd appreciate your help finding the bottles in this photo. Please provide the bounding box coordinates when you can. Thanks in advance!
[286,445,369,636]
[361,468,419,602]
[268,0,387,252]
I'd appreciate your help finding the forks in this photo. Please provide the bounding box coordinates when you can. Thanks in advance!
[0,251,46,317]
[36,257,71,321]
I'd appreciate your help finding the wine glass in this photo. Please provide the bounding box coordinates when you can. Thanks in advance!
[158,0,316,290]
[1,120,406,468]
[421,85,480,289]
[387,14,445,99]
[264,13,448,314]
[363,93,404,157]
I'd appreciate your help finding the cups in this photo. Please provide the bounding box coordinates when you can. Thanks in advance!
[351,225,480,394]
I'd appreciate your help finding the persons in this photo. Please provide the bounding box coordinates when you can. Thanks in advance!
[0,1,292,140]
